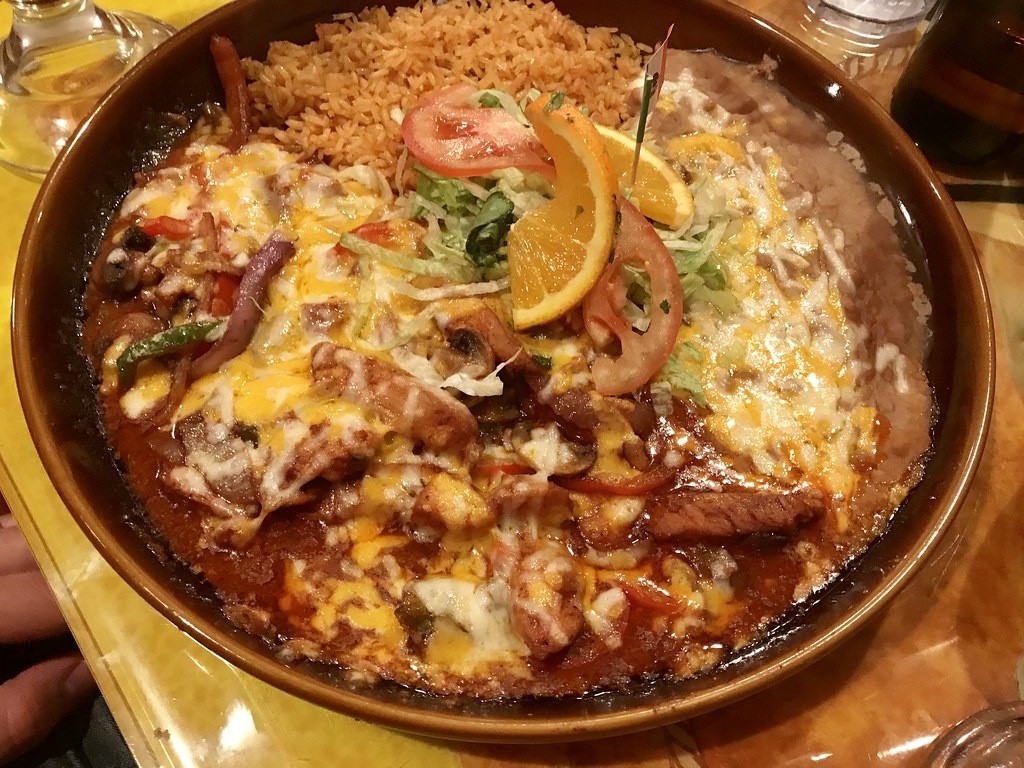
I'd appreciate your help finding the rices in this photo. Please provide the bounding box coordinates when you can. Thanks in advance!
[240,0,660,192]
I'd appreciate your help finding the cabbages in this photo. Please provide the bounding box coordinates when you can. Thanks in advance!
[338,88,738,399]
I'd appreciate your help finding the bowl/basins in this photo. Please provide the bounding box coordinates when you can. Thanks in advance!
[11,0,997,743]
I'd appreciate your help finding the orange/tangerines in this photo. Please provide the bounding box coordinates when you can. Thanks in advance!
[505,92,697,330]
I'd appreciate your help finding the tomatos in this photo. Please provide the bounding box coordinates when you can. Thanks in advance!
[399,90,558,178]
[583,198,684,396]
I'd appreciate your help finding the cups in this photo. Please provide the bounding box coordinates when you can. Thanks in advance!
[890,0,1024,177]
[799,0,938,57]
[923,701,1024,768]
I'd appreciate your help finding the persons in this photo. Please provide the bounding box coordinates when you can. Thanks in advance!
[0,515,96,768]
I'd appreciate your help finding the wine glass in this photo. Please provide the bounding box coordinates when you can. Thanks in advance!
[0,0,178,184]
